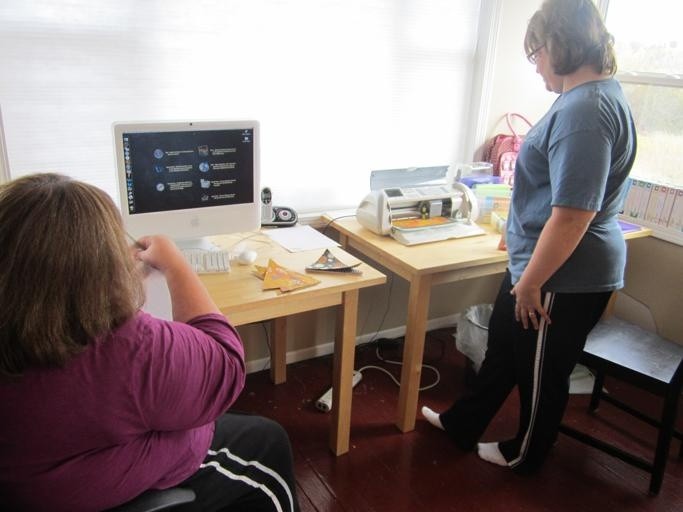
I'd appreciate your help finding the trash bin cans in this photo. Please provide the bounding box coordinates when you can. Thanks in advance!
[466,304,494,376]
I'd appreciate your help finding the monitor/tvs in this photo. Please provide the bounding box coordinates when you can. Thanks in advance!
[112,121,260,249]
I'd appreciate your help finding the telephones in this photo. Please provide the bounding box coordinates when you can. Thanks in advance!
[261,188,298,226]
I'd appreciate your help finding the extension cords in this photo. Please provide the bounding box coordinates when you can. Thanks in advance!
[315,369,363,413]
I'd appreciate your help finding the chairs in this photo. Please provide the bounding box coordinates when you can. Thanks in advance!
[109,485,197,511]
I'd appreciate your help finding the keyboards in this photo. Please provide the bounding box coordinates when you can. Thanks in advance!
[179,249,231,274]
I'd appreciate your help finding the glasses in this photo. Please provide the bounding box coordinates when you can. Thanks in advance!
[527,44,546,63]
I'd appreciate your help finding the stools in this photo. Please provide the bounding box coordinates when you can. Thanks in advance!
[559,313,683,495]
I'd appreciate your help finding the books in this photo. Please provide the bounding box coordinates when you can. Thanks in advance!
[617,218,640,233]
[619,177,683,234]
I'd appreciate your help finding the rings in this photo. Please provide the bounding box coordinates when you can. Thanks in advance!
[527,310,535,316]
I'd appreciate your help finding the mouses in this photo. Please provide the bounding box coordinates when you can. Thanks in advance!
[239,250,257,264]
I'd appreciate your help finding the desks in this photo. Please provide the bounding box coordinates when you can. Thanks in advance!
[182,222,385,456]
[321,213,652,432]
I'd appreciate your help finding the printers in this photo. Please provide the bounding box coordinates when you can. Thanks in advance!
[356,166,487,247]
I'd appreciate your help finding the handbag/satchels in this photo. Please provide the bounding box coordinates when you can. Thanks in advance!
[483,112,532,176]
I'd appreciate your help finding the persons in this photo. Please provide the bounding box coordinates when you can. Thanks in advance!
[418,0,638,474]
[0,171,301,512]
